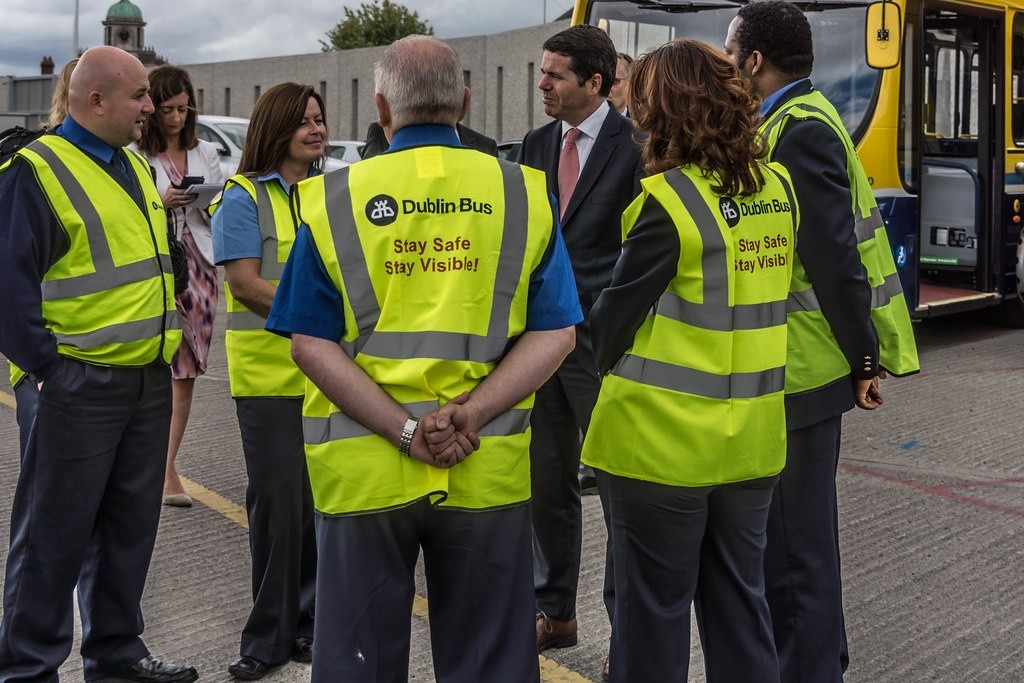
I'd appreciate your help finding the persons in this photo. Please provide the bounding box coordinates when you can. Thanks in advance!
[582,39,802,683]
[219,79,330,679]
[291,33,577,683]
[0,44,225,683]
[724,0,888,683]
[362,121,499,162]
[514,24,645,679]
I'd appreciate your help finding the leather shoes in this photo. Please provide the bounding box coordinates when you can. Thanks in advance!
[228,651,290,679]
[287,634,314,663]
[535,607,577,655]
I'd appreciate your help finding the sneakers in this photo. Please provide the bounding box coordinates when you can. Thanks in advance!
[85,650,199,683]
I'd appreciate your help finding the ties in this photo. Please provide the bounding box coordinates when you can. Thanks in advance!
[557,128,583,222]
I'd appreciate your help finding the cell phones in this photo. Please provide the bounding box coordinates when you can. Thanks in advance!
[178,174,205,190]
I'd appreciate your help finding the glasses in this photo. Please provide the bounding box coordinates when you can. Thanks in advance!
[613,76,630,86]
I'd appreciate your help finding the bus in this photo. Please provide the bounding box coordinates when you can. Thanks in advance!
[569,0,1024,336]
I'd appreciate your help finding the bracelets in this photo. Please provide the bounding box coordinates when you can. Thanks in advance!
[398,415,421,458]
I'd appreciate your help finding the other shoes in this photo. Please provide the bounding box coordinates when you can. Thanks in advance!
[163,493,192,507]
[579,472,600,495]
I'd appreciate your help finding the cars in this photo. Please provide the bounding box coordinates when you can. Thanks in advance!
[195,115,350,174]
[324,140,365,162]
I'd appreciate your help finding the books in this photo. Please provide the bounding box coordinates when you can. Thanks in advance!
[181,183,225,209]
